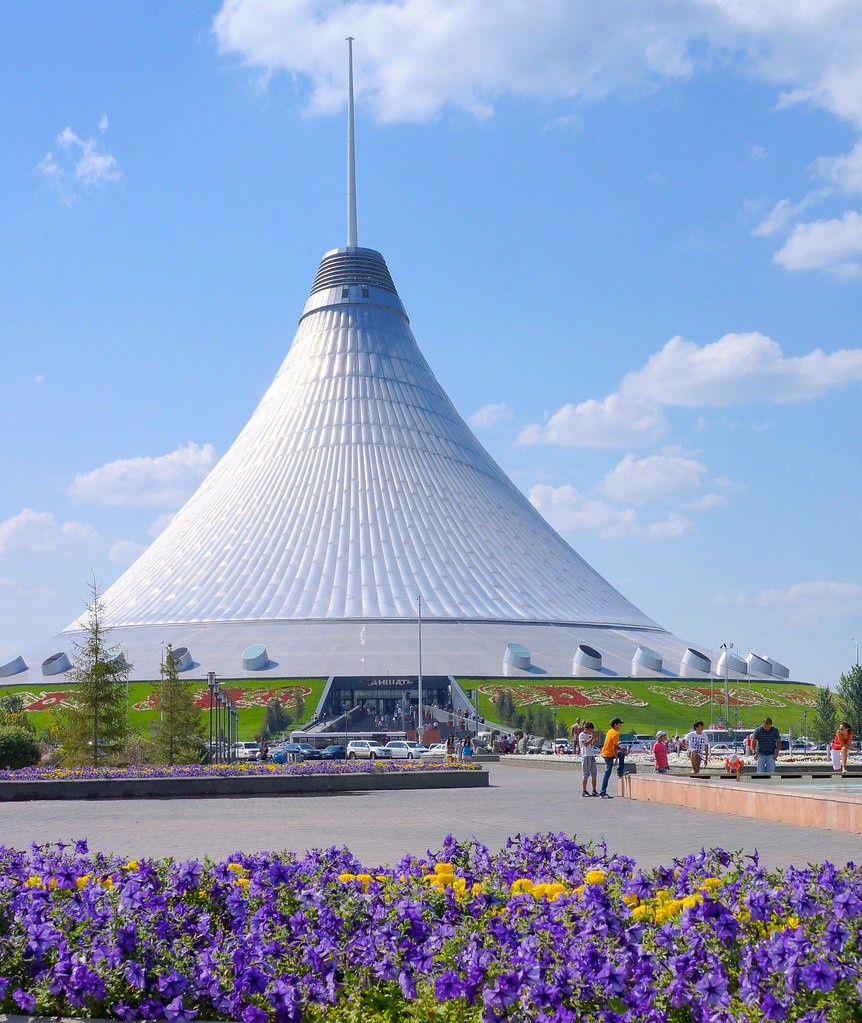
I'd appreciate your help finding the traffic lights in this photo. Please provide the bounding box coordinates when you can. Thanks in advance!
[467,688,471,699]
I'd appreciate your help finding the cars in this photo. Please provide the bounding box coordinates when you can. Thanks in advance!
[614,733,862,753]
[555,738,569,752]
[346,740,394,760]
[207,740,286,761]
[384,739,431,760]
[428,742,445,754]
[285,742,321,760]
[318,744,347,760]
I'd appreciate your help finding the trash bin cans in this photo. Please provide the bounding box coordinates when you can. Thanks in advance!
[616,763,636,774]
[286,749,301,763]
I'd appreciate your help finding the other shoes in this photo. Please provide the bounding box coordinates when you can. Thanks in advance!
[844,768,847,772]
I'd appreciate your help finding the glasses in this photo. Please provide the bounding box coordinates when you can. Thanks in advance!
[839,729,844,731]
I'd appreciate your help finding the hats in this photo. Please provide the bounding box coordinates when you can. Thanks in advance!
[466,735,470,738]
[656,731,667,739]
[610,718,624,726]
[449,733,453,736]
[730,754,738,762]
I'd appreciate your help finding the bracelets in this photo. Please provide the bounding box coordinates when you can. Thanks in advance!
[753,752,756,755]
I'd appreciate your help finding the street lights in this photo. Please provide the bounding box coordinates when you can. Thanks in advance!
[416,595,423,746]
[732,706,739,753]
[849,636,859,666]
[552,706,557,756]
[201,671,222,765]
[213,680,240,765]
[802,708,807,754]
[719,642,734,730]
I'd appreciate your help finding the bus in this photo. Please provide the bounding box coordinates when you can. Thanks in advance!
[701,728,759,752]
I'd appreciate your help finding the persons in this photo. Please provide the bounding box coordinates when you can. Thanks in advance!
[831,723,859,773]
[653,731,671,774]
[255,747,270,759]
[750,717,782,773]
[725,755,744,782]
[461,735,474,759]
[445,733,456,755]
[578,721,599,797]
[347,715,353,727]
[598,717,630,799]
[315,712,318,721]
[432,721,438,729]
[457,737,463,755]
[571,718,586,754]
[680,720,709,773]
[499,734,534,755]
[668,735,687,753]
[558,744,568,754]
[743,734,756,755]
[323,712,328,725]
[362,702,468,732]
[472,710,476,721]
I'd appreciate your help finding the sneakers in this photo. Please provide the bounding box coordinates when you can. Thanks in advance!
[582,791,591,796]
[618,771,629,779]
[592,791,602,797]
[600,794,613,799]
